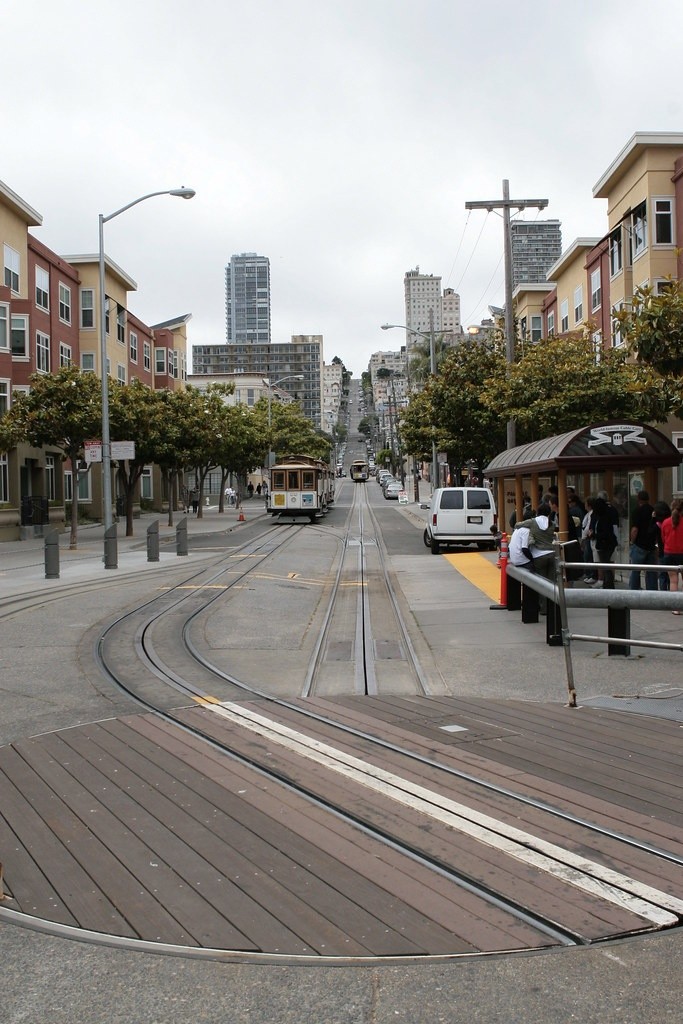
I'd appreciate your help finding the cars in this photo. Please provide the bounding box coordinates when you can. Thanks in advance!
[376,470,404,499]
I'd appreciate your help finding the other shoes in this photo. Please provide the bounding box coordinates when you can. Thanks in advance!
[585,578,596,583]
[590,580,603,587]
[584,578,588,581]
[671,611,680,615]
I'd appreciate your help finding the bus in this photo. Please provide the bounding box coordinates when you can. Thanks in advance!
[350,459,369,483]
[265,454,335,524]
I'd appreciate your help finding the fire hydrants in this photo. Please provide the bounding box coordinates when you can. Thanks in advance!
[206,497,210,505]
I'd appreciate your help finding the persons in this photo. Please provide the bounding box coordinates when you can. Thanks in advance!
[224,487,236,505]
[180,485,190,513]
[629,491,683,615]
[262,481,268,495]
[508,484,620,616]
[190,485,200,513]
[256,483,261,495]
[247,481,254,498]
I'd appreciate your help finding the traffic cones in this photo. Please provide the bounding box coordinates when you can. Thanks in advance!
[237,506,246,521]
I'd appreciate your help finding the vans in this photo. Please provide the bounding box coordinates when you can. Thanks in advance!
[420,487,497,554]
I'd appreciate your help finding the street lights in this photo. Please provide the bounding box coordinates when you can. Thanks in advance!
[381,323,438,489]
[99,187,196,559]
[268,374,305,481]
